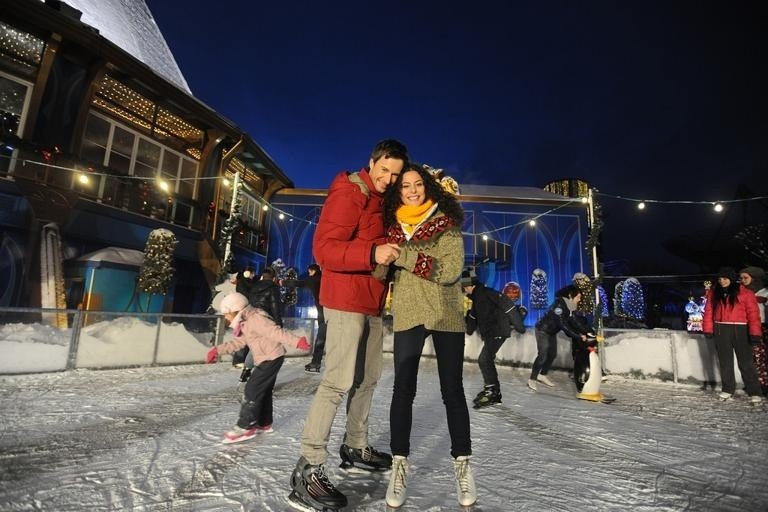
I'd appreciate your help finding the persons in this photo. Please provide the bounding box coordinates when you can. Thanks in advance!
[238,267,283,382]
[232,266,264,369]
[739,265,767,399]
[289,139,410,511]
[460,271,526,412]
[373,163,477,507]
[702,268,763,411]
[205,292,311,444]
[526,285,596,390]
[278,264,327,375]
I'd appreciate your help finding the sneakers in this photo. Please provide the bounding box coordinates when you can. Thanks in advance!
[290,456,348,509]
[537,374,553,386]
[305,363,320,369]
[225,425,272,439]
[473,388,502,405]
[527,379,537,390]
[719,391,731,398]
[454,456,477,506]
[340,444,407,507]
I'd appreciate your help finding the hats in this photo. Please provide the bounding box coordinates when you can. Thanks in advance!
[220,293,248,313]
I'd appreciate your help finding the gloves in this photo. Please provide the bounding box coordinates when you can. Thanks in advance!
[207,348,217,363]
[299,336,311,349]
